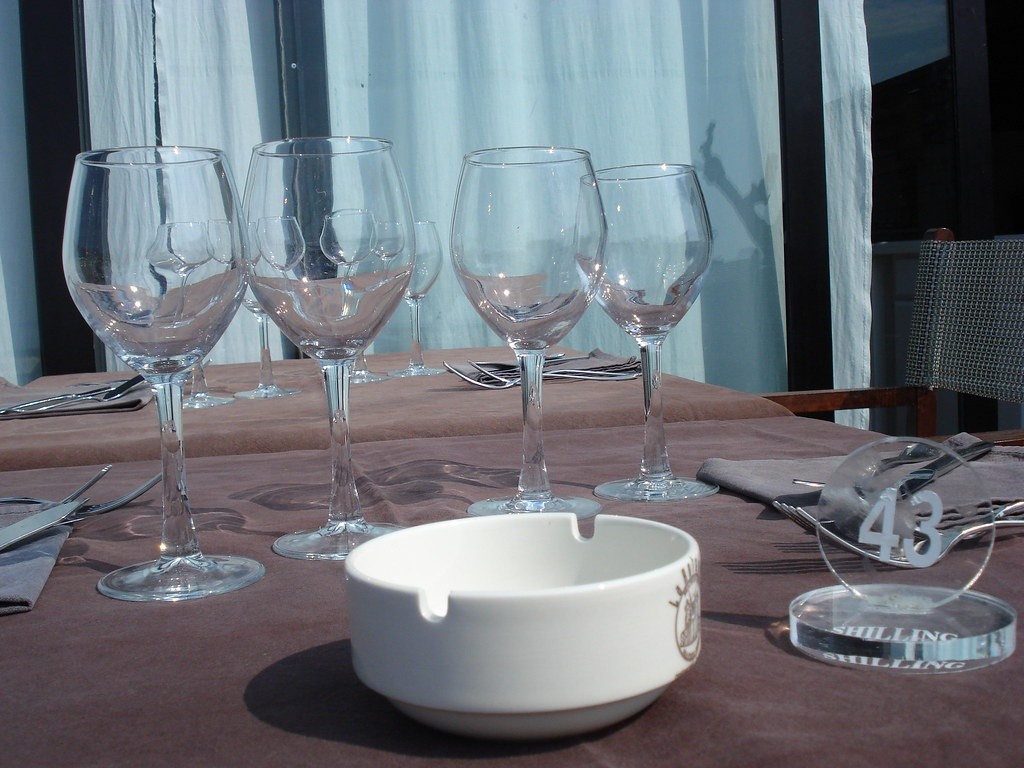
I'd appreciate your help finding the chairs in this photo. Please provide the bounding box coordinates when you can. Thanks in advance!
[759,228,1024,446]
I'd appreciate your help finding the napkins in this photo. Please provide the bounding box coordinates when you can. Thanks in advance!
[695,431,1024,529]
[448,347,643,385]
[0,503,76,617]
[0,376,153,420]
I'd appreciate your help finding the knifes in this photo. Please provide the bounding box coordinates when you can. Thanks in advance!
[0,497,92,551]
[858,441,994,507]
[0,387,116,413]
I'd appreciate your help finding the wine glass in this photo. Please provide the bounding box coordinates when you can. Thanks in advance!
[60,135,718,602]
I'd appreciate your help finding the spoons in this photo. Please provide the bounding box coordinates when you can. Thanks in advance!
[6,374,147,410]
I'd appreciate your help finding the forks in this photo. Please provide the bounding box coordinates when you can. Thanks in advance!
[0,465,162,523]
[771,440,1024,568]
[443,352,642,390]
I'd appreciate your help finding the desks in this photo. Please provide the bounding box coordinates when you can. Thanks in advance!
[0,416,1024,768]
[0,343,795,472]
